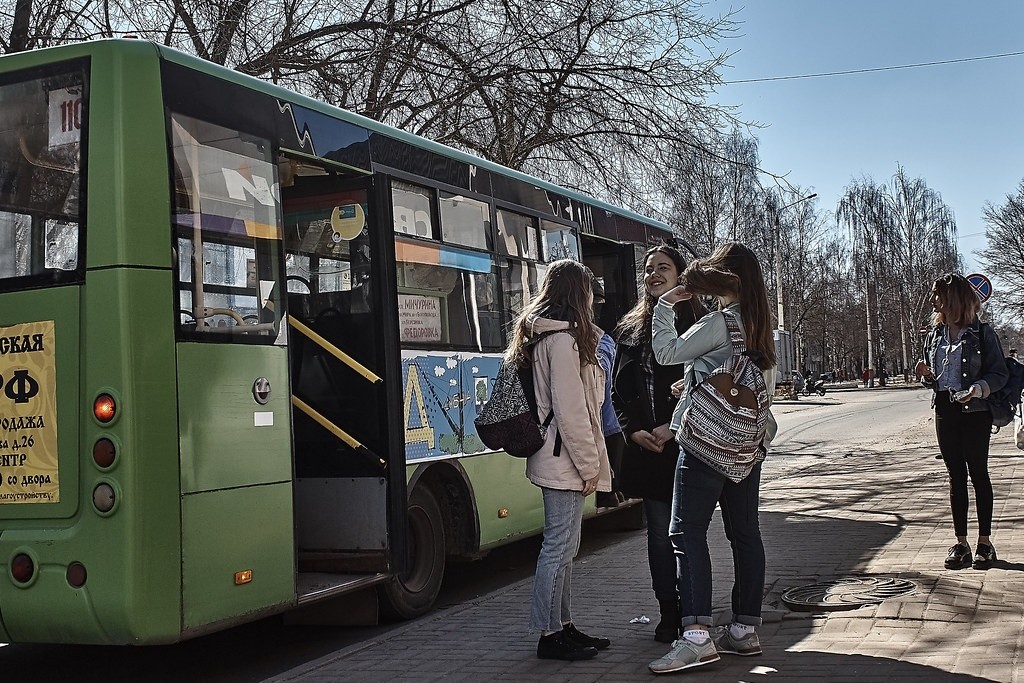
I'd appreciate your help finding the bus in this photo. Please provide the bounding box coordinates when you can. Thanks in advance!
[0,35,700,645]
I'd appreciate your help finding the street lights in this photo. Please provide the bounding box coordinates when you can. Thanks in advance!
[774,194,818,380]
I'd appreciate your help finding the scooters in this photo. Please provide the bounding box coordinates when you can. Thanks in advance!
[801,370,828,396]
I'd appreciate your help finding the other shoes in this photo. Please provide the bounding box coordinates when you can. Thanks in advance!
[945,542,972,569]
[972,541,997,569]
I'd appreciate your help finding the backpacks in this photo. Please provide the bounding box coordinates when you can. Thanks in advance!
[980,323,1024,432]
[675,311,770,485]
[474,327,582,459]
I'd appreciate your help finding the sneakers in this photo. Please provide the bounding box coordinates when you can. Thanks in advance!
[562,626,610,649]
[648,637,722,674]
[537,630,599,660]
[713,625,762,656]
[652,603,680,641]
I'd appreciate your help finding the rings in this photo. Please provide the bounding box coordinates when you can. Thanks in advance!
[592,485,595,488]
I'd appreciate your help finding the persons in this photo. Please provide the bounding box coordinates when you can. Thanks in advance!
[594,333,628,507]
[611,235,710,643]
[502,258,613,659]
[914,272,1008,569]
[646,242,777,673]
[862,367,868,388]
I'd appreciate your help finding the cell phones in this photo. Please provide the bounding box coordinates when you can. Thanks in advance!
[955,390,972,401]
